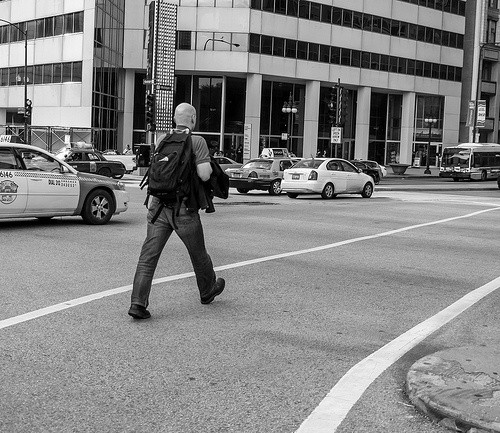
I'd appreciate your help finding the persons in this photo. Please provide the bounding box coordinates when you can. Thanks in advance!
[316,149,323,158]
[214,145,242,164]
[74,153,80,161]
[128,103,225,319]
[123,145,131,155]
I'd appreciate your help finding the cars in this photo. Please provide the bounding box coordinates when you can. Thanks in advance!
[31,147,125,180]
[379,165,387,177]
[224,148,299,195]
[349,160,380,183]
[55,142,138,173]
[280,154,375,199]
[0,135,130,224]
[214,157,242,172]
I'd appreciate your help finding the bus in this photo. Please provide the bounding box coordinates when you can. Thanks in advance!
[439,142,500,181]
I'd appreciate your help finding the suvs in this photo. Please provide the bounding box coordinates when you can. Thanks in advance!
[360,160,383,180]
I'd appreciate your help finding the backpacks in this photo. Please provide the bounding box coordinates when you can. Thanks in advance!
[148,128,192,200]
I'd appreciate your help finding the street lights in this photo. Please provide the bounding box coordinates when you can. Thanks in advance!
[282,91,298,150]
[203,37,240,51]
[424,118,437,174]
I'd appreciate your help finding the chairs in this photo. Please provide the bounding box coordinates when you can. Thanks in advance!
[329,163,338,170]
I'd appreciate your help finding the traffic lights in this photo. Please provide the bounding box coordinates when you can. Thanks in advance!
[338,88,349,125]
[146,94,154,123]
[327,88,336,125]
[25,99,32,118]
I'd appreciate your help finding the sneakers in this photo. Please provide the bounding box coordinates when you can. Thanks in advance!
[128,304,150,319]
[201,278,226,303]
